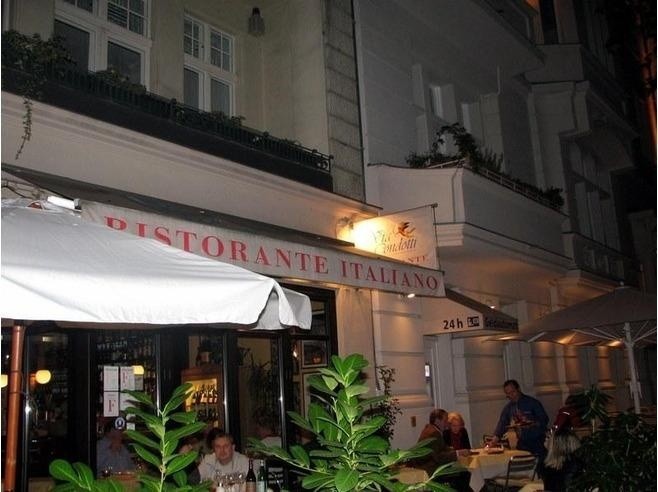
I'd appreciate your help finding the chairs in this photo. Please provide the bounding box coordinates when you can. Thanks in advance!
[505,454,540,492]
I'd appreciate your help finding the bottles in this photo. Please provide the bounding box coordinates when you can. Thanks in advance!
[256,459,267,492]
[246,459,256,492]
[191,384,218,428]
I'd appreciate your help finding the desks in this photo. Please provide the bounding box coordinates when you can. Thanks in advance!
[382,468,426,491]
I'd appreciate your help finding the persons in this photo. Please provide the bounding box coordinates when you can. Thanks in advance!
[483,378,553,491]
[405,407,471,492]
[180,405,328,491]
[96,416,136,480]
[443,412,472,452]
[551,393,593,439]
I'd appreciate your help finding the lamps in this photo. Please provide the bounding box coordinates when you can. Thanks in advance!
[247,7,266,37]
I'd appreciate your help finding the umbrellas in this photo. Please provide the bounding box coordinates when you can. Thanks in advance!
[0,193,314,492]
[479,279,656,429]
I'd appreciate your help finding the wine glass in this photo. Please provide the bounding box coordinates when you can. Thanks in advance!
[214,470,246,491]
